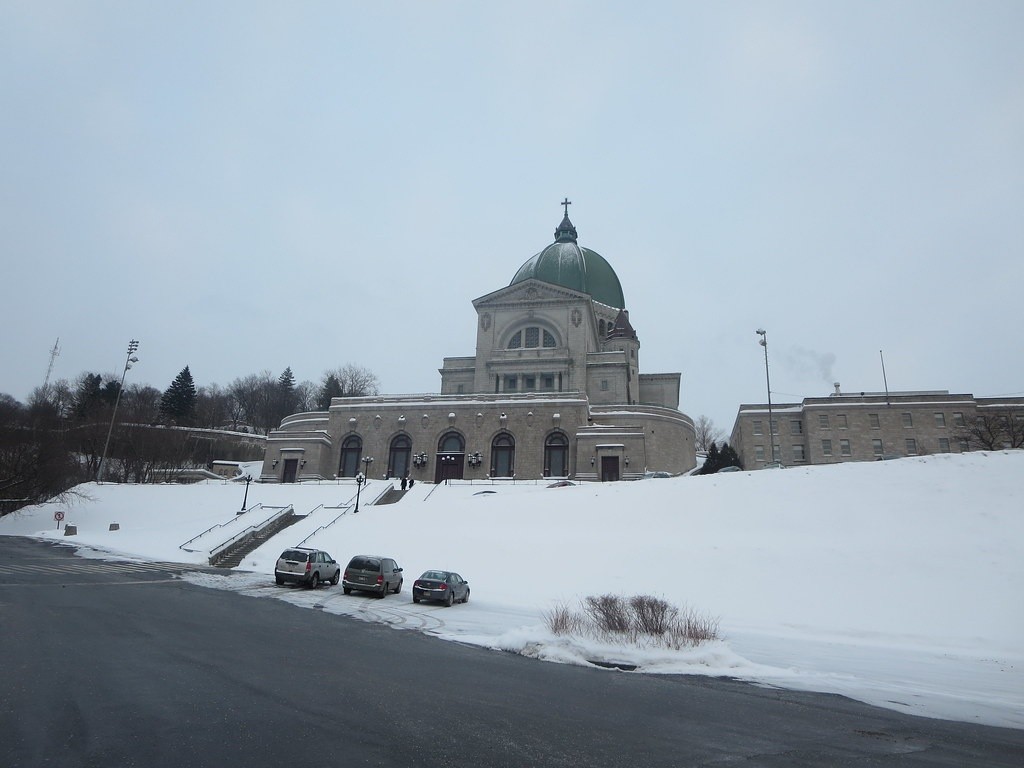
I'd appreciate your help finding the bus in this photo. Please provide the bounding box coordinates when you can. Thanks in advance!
[342,555,403,599]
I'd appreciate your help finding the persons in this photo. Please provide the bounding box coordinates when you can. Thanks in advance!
[401,478,407,490]
[409,478,414,488]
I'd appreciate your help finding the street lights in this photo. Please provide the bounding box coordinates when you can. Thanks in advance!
[442,456,455,485]
[361,457,374,485]
[240,475,252,510]
[96,338,139,483]
[756,328,775,462]
[354,472,365,513]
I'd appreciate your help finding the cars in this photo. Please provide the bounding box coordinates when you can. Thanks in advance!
[413,569,470,607]
[764,459,786,469]
[652,471,682,478]
[717,466,742,473]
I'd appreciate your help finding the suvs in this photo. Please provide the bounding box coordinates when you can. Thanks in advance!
[275,546,340,589]
